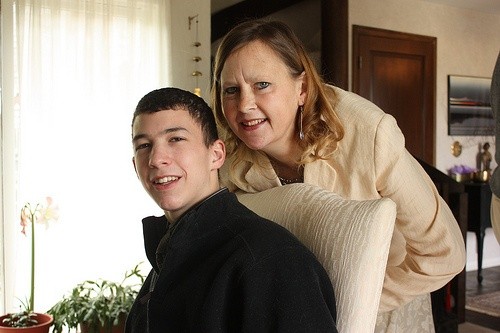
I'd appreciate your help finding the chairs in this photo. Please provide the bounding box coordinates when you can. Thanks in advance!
[235,182,397,332]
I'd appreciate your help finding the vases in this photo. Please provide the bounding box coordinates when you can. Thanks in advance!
[0,310,54,333]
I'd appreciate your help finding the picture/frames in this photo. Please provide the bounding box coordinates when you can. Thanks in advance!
[448,74,497,135]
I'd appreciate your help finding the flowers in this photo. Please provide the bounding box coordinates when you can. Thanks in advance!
[20,196,52,312]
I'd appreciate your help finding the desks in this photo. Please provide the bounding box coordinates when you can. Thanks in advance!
[462,180,494,285]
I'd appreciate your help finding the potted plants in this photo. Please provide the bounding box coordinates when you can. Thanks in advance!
[49,262,148,333]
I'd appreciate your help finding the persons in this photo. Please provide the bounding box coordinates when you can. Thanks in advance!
[123,88,338,333]
[490,51,500,245]
[200,17,466,333]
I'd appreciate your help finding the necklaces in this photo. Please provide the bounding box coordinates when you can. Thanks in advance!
[278,176,302,182]
